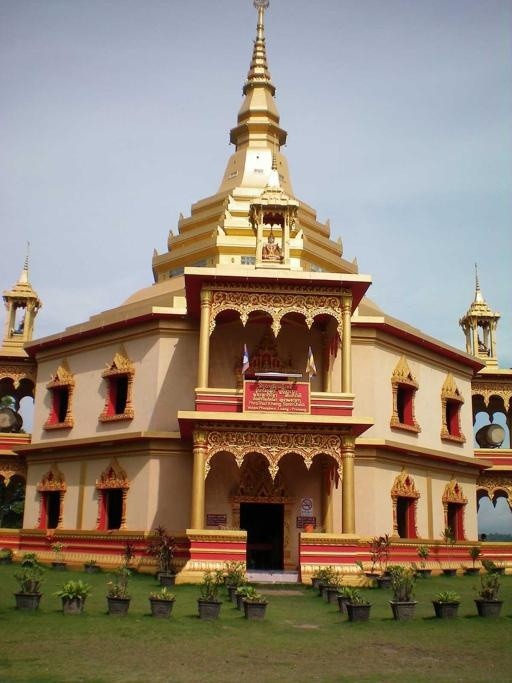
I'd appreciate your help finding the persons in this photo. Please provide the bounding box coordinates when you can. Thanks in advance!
[262,232,283,259]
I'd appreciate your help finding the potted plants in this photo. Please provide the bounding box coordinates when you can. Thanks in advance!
[310,526,506,622]
[0,522,270,621]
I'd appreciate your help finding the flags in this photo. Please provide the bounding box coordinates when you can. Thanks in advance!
[241,344,250,375]
[306,344,317,378]
